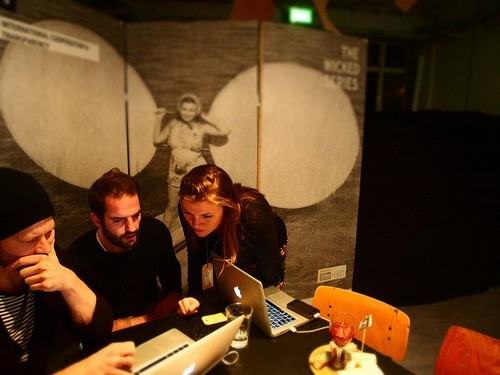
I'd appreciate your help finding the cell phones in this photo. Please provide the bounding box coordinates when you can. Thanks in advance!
[287,299,320,319]
[201,312,227,325]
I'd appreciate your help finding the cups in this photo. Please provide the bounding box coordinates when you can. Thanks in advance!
[226,302,253,350]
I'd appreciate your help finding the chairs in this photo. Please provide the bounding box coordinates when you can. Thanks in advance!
[434,326,500,375]
[312,286,410,363]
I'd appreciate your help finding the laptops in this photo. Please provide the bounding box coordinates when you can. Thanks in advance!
[210,252,316,337]
[122,315,245,375]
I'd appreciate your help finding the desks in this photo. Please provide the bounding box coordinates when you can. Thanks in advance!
[34,296,416,375]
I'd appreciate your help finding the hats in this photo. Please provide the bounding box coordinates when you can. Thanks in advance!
[0,167,55,242]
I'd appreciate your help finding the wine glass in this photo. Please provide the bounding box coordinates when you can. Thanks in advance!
[329,312,355,369]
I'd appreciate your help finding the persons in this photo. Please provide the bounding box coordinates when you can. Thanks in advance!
[178,164,287,315]
[0,168,136,375]
[62,169,182,325]
[154,94,229,232]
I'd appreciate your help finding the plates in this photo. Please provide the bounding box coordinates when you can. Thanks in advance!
[308,344,354,375]
